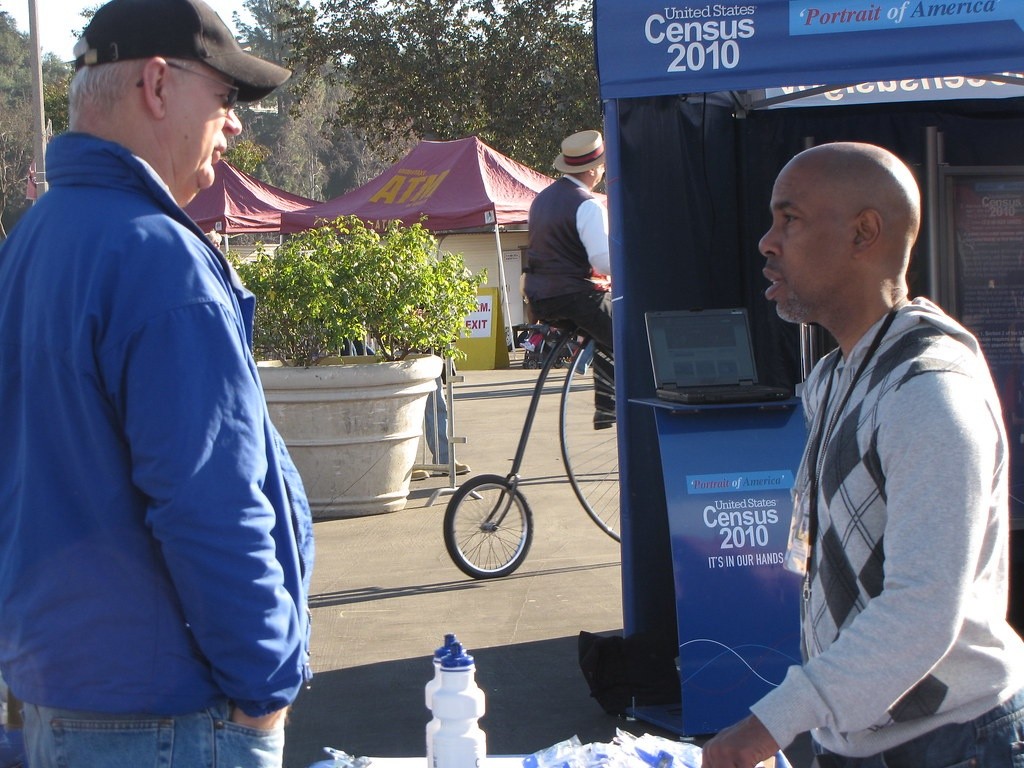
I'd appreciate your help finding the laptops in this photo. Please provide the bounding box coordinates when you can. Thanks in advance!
[644,308,791,406]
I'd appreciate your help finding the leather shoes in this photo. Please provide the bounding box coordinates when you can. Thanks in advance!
[594,410,616,429]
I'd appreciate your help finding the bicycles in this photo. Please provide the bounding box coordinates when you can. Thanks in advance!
[437,299,624,580]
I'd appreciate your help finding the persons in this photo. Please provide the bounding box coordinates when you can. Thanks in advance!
[521,129,617,431]
[202,229,222,251]
[699,141,1024,768]
[0,2,315,766]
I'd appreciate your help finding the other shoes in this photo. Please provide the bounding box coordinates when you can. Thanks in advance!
[412,469,430,480]
[433,459,471,474]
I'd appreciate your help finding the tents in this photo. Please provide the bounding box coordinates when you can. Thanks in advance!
[589,0,1024,737]
[279,135,606,361]
[178,158,321,262]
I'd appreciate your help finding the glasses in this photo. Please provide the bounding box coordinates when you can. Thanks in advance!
[137,61,239,107]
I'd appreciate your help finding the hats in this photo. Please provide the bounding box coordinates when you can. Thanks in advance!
[75,0,292,102]
[554,129,605,173]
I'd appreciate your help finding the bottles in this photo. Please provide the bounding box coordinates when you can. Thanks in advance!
[432,641,487,768]
[423,634,469,766]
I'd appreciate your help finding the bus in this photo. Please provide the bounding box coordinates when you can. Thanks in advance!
[591,1,1022,756]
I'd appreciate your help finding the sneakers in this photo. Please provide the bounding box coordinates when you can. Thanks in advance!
[520,341,534,352]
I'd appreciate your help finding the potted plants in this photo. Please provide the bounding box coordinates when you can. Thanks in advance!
[242,217,484,518]
